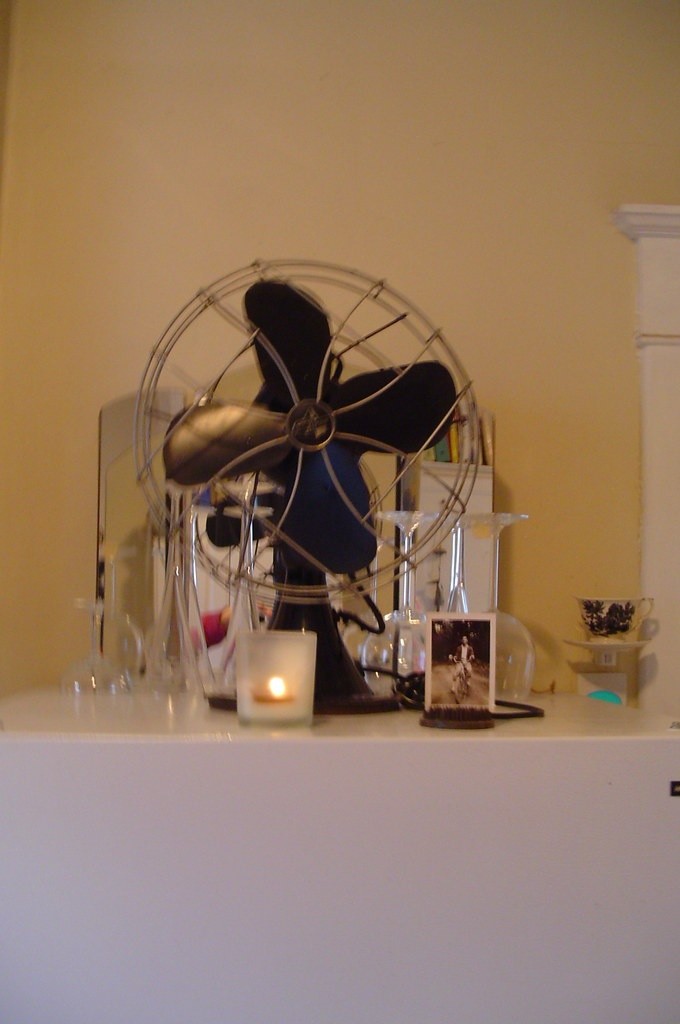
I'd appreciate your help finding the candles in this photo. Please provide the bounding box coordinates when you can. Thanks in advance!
[233,631,319,731]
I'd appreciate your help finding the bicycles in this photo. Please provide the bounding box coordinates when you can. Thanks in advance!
[449,655,474,704]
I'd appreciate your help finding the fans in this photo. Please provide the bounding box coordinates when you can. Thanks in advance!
[132,256,481,717]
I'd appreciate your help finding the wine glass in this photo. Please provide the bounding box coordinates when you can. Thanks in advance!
[58,469,540,733]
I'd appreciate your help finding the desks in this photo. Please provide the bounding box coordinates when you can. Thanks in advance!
[2,686,680,1024]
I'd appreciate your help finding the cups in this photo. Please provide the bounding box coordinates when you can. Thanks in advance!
[572,592,655,639]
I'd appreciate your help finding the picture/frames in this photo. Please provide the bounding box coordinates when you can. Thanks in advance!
[425,611,497,717]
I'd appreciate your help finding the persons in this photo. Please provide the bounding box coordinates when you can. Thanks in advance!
[449,636,475,696]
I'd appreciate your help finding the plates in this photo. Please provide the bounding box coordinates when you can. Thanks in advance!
[560,636,652,653]
